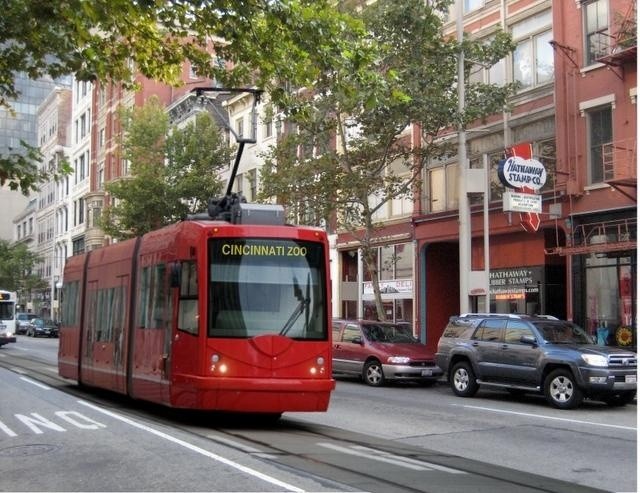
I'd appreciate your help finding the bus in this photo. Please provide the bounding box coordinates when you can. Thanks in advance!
[0,290,20,347]
[56,87,336,426]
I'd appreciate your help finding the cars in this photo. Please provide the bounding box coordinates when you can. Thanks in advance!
[16,312,59,338]
[332,320,444,387]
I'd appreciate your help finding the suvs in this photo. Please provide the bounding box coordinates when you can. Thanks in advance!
[434,312,637,410]
